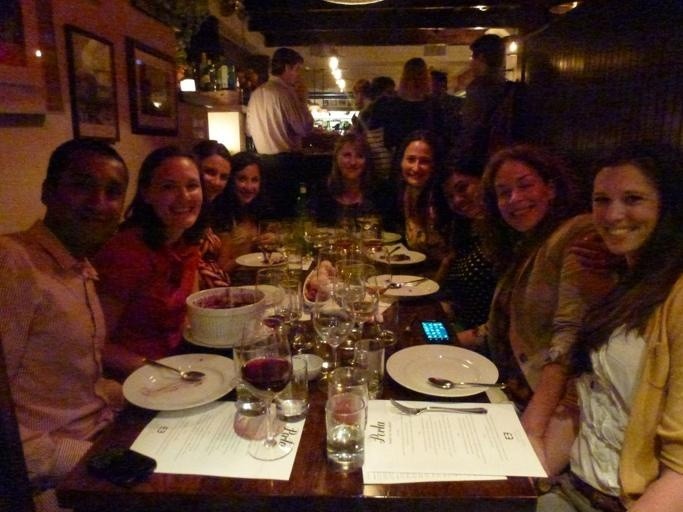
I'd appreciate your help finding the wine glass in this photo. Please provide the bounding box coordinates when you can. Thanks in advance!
[244,212,384,471]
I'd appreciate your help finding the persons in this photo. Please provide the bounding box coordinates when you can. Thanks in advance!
[87,144,211,393]
[378,130,446,255]
[0,133,128,512]
[431,157,517,335]
[531,147,681,510]
[240,48,316,225]
[348,34,517,226]
[225,151,266,269]
[308,127,393,229]
[186,137,233,287]
[455,135,619,487]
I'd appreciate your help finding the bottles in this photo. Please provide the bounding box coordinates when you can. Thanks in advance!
[176,44,242,95]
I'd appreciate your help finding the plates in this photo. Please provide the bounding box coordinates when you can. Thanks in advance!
[120,241,289,413]
[360,231,499,399]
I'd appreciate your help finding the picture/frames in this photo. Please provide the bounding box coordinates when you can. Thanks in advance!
[124,32,180,139]
[63,22,120,144]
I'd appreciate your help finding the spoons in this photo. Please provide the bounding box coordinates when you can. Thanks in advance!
[428,378,508,393]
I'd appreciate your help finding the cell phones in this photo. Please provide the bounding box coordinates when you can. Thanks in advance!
[86,445,157,484]
[421,319,450,343]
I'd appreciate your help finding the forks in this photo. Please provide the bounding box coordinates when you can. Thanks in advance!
[388,399,486,420]
